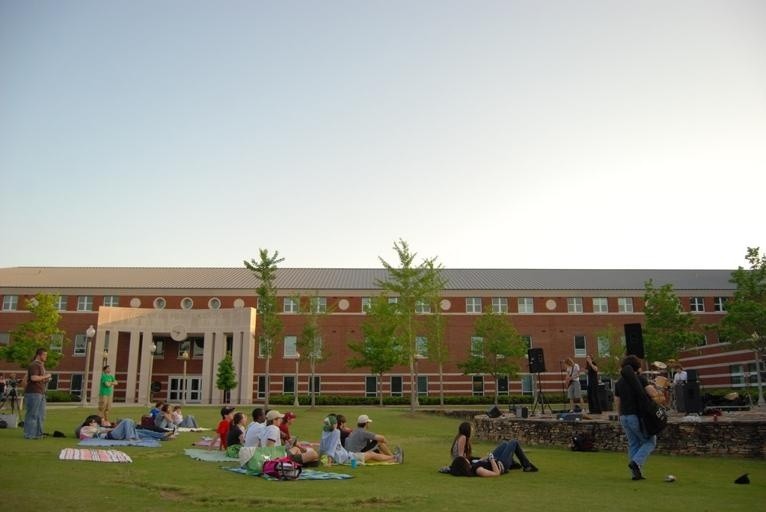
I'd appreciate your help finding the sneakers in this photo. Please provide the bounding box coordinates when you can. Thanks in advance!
[509,463,522,469]
[629,461,645,480]
[392,445,403,464]
[524,462,538,472]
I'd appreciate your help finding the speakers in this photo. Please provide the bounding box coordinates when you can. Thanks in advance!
[624,323,645,360]
[516,407,528,417]
[486,405,502,418]
[527,347,546,373]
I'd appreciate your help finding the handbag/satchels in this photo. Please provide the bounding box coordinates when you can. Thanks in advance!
[263,457,302,480]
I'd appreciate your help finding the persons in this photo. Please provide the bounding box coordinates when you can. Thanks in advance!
[654,372,668,390]
[81,417,175,440]
[208,406,320,464]
[451,422,480,463]
[614,356,656,480]
[0,349,52,440]
[320,413,402,465]
[150,403,196,429]
[451,439,538,477]
[673,364,688,384]
[98,365,118,420]
[585,354,601,414]
[564,358,584,413]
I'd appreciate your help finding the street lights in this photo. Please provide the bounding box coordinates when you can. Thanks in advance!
[182,351,188,400]
[104,350,108,367]
[147,342,157,402]
[80,325,96,400]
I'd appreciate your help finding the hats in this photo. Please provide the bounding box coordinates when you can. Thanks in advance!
[284,412,296,420]
[221,406,235,415]
[324,416,337,424]
[266,410,284,420]
[358,415,372,424]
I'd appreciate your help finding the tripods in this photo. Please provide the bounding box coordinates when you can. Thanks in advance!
[0,389,21,421]
[532,374,553,414]
